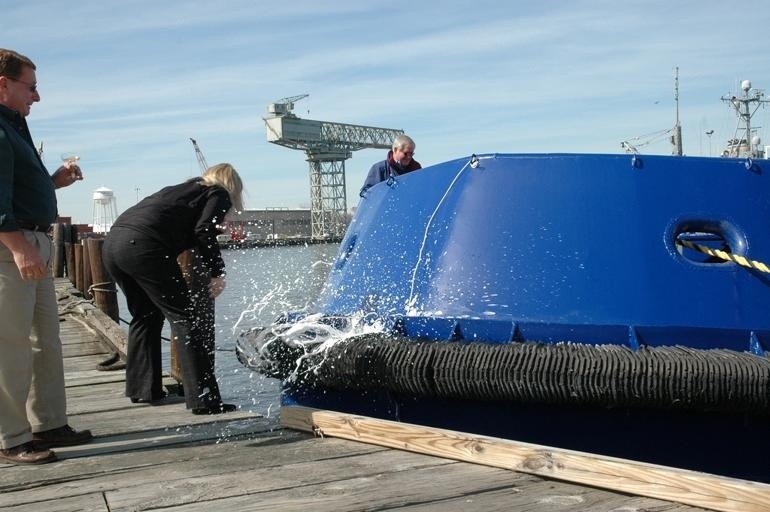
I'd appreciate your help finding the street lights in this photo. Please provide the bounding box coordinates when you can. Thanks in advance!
[133,187,142,204]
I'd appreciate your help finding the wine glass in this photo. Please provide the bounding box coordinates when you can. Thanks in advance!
[60,152,81,181]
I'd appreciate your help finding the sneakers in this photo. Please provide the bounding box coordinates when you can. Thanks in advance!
[130,391,167,403]
[192,402,237,415]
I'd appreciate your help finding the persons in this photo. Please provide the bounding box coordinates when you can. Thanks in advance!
[361,134,422,196]
[0,48,92,465]
[100,161,244,415]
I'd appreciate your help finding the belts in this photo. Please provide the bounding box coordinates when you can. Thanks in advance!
[15,219,56,233]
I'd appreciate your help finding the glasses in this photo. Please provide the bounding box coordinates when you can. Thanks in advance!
[398,149,415,158]
[6,76,38,93]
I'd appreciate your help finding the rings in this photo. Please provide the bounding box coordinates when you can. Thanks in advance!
[27,273,33,276]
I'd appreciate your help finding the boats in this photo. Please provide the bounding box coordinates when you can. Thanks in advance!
[236,66,769,485]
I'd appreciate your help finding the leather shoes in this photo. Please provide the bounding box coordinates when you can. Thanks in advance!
[0,441,57,465]
[32,423,89,446]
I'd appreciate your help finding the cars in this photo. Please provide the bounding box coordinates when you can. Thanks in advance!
[216,231,333,242]
